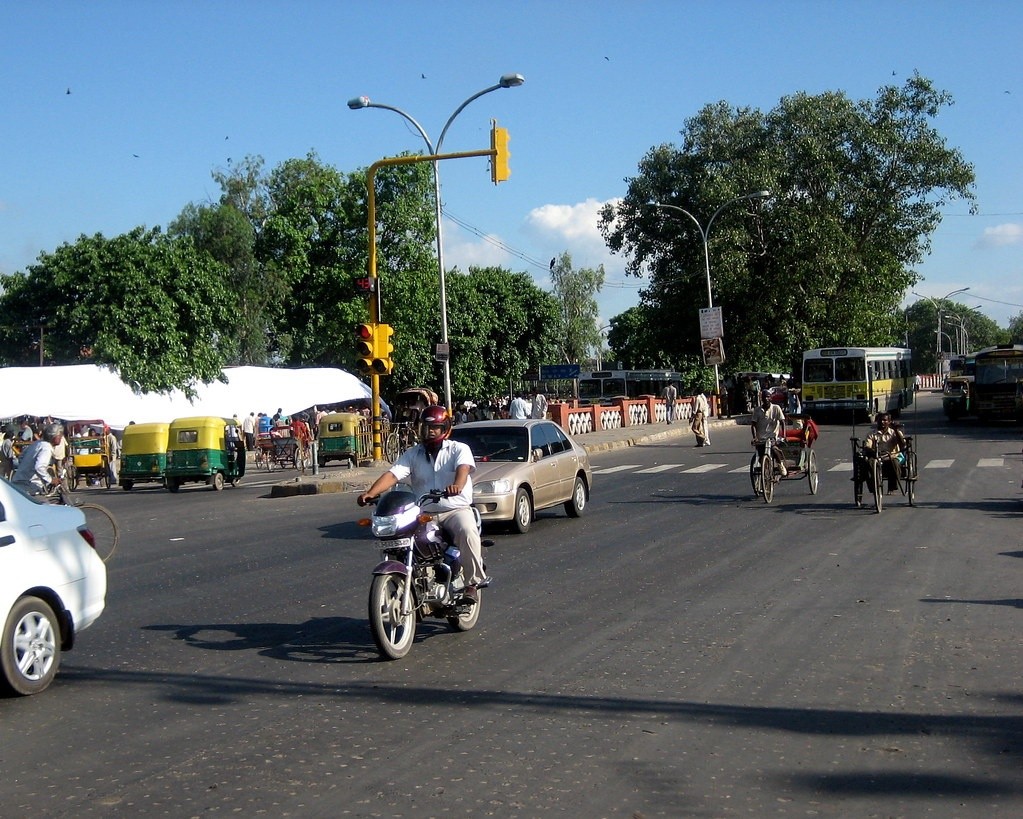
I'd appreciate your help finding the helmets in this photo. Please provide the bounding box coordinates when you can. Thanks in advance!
[420,406,451,448]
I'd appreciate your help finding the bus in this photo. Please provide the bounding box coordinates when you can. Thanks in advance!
[802,346,914,424]
[735,372,790,391]
[949,352,997,393]
[577,369,682,415]
[970,344,1023,426]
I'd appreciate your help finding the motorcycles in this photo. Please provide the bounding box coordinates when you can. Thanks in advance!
[942,375,975,424]
[164,416,246,493]
[317,412,369,468]
[356,488,497,661]
[118,422,171,491]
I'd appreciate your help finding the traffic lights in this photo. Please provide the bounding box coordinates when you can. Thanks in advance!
[490,118,512,186]
[355,322,391,376]
[353,276,376,293]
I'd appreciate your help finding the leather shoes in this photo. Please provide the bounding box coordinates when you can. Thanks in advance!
[463,585,479,603]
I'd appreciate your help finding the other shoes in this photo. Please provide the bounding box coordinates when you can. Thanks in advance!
[869,486,875,493]
[777,462,787,476]
[700,438,705,444]
[694,444,703,447]
[888,484,898,491]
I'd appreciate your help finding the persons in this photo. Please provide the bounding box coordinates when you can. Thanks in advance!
[0,418,117,504]
[866,412,907,495]
[688,388,711,447]
[720,373,786,415]
[750,391,787,476]
[129,421,135,425]
[660,379,677,424]
[233,406,370,461]
[358,406,487,603]
[789,388,803,428]
[914,373,921,391]
[454,386,548,425]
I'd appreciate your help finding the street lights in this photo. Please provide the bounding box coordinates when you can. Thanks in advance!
[346,73,526,417]
[645,190,771,420]
[596,322,619,371]
[911,287,982,375]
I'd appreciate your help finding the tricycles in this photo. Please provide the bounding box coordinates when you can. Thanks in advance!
[750,414,820,504]
[384,387,439,465]
[254,424,313,473]
[67,420,112,492]
[849,420,920,515]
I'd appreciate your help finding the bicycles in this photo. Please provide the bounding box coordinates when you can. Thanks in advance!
[23,467,121,564]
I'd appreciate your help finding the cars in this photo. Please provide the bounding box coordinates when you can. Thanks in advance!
[0,477,108,696]
[767,385,789,409]
[447,419,593,535]
